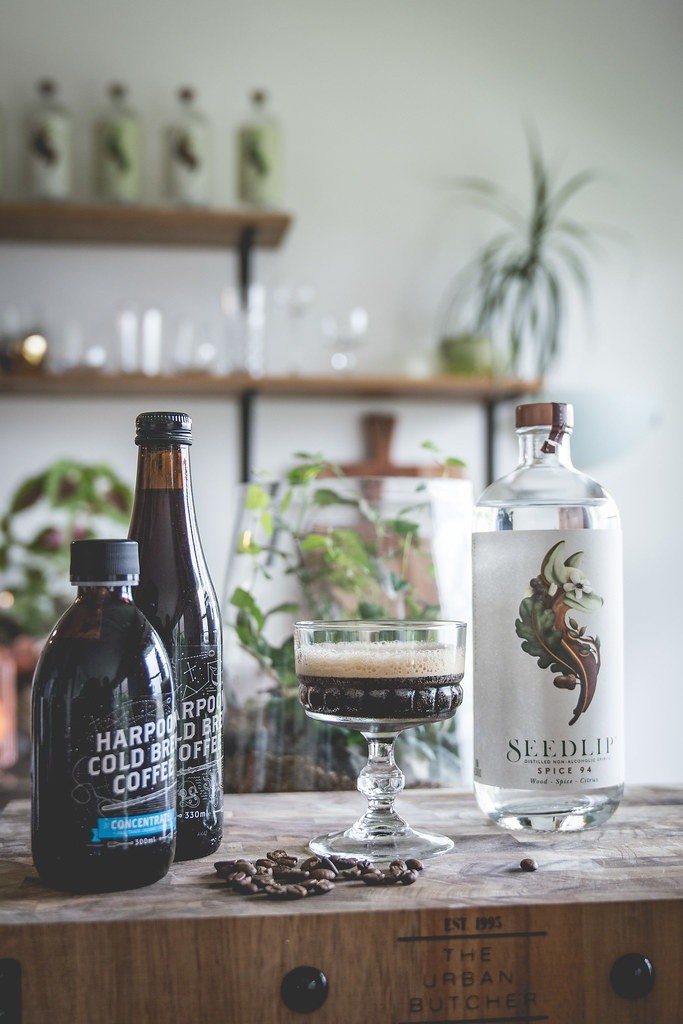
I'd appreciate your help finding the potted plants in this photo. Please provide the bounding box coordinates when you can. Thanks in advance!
[435,121,619,385]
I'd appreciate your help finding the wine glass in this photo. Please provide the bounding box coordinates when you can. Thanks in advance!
[292,618,468,864]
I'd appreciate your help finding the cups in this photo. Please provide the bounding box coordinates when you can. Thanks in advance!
[120,310,160,371]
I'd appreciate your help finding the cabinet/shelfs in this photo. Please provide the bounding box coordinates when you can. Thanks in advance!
[0,198,531,485]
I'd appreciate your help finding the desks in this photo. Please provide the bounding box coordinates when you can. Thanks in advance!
[0,787,683,1023]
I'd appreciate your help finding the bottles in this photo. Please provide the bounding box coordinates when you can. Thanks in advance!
[23,78,71,202]
[31,539,175,895]
[88,81,139,206]
[123,412,225,863]
[159,88,211,206]
[234,90,279,208]
[471,402,628,833]
